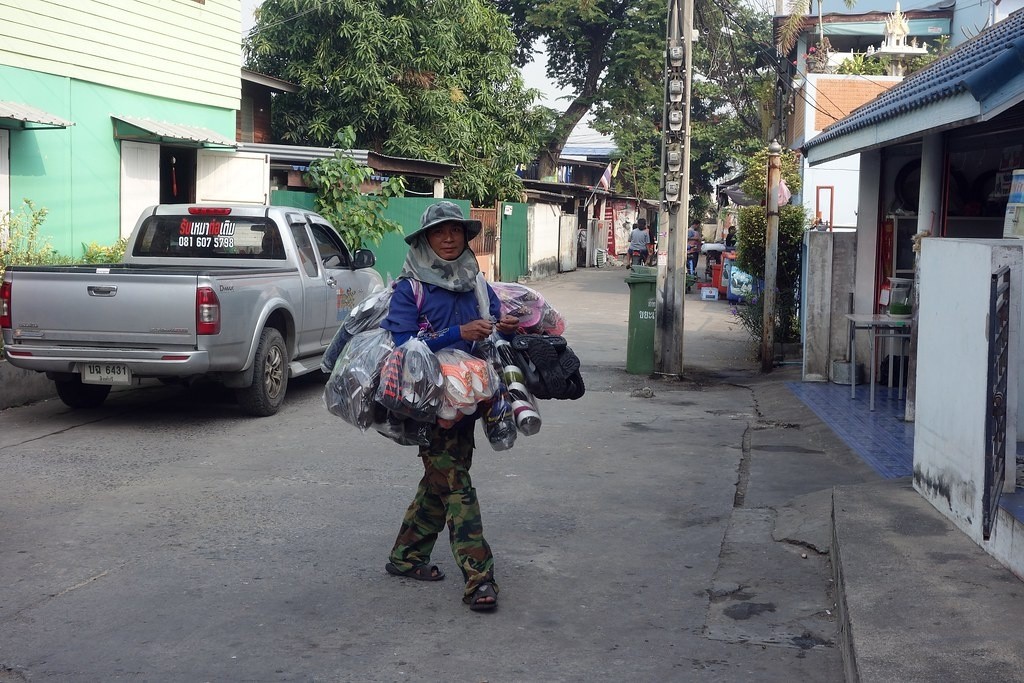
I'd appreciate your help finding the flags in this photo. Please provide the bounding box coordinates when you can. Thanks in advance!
[612,161,620,177]
[601,163,611,191]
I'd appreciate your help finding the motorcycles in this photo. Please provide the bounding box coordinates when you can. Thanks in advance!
[630,249,645,272]
[686,245,698,294]
[707,249,718,277]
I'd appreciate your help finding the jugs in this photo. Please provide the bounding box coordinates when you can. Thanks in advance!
[887,277,913,314]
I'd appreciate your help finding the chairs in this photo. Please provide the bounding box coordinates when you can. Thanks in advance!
[153,232,288,260]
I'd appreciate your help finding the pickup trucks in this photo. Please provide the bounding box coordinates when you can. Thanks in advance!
[0,204,385,417]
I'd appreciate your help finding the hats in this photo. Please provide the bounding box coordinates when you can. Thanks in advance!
[398,201,482,293]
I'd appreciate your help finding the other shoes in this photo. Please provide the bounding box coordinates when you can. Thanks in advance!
[320,282,586,452]
[626,265,630,269]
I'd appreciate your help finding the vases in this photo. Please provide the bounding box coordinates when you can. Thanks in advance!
[805,61,825,73]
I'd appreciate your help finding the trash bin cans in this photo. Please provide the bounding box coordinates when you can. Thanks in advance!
[624,263,698,376]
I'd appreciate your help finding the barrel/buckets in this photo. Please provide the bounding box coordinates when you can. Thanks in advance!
[712,265,723,288]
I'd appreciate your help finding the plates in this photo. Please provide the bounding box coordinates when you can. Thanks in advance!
[886,311,890,313]
[887,313,912,318]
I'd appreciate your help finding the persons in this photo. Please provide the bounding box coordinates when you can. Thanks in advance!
[687,219,705,274]
[723,264,730,279]
[726,226,737,247]
[732,272,751,288]
[380,200,519,610]
[626,219,654,269]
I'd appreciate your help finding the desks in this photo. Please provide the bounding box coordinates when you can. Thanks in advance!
[843,313,912,411]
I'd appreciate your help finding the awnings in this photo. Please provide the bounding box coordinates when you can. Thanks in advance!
[0,101,76,131]
[111,116,243,149]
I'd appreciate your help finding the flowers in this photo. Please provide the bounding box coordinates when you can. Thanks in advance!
[793,37,832,71]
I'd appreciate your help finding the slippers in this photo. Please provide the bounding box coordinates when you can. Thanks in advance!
[385,561,446,581]
[470,583,498,611]
